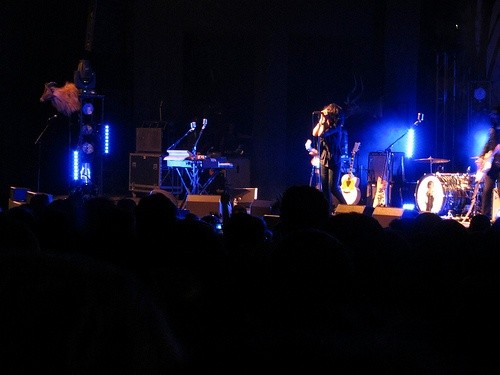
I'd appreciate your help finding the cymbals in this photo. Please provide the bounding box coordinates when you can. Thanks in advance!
[414,158,450,163]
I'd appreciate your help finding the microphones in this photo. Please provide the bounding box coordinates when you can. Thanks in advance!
[202,119,207,130]
[314,111,323,114]
[189,122,196,132]
[414,113,424,125]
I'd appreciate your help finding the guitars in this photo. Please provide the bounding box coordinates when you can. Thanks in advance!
[372,148,393,207]
[475,143,500,183]
[340,141,362,204]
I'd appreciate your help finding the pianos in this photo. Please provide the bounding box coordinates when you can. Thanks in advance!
[163,149,234,219]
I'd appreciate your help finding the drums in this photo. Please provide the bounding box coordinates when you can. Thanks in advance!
[413,172,474,217]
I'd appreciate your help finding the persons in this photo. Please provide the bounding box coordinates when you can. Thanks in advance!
[480,111,500,218]
[0,180,500,375]
[305,136,322,190]
[312,103,346,204]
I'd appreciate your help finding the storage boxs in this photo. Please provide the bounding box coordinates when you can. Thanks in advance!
[234,188,258,203]
[186,195,223,219]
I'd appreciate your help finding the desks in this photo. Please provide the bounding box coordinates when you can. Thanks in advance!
[167,159,233,211]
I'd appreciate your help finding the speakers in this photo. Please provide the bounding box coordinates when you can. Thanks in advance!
[367,152,405,183]
[227,187,274,217]
[335,204,404,228]
[136,128,162,153]
[186,195,222,218]
